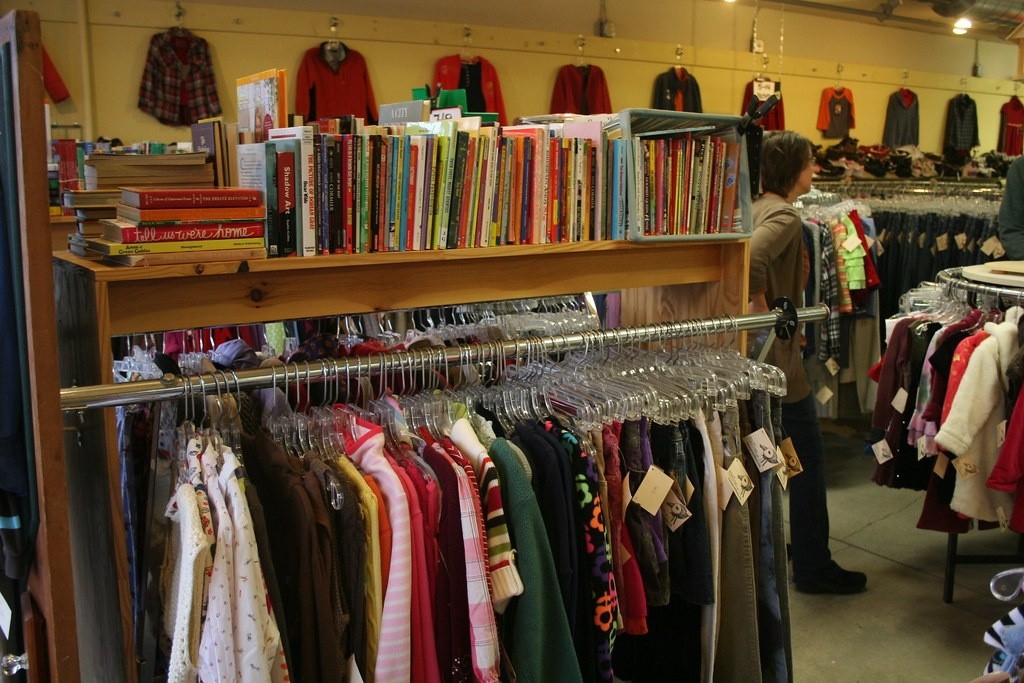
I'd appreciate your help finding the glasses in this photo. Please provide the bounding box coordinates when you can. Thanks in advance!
[807,156,816,166]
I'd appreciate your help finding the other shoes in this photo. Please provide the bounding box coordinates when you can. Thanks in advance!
[798,560,868,595]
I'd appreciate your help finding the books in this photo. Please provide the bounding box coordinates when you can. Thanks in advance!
[46,68,740,268]
[986,261,1024,276]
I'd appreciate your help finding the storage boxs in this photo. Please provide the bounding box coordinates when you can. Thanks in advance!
[603,104,755,245]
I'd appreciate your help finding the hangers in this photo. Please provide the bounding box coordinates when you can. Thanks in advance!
[797,179,1006,222]
[112,294,789,511]
[174,9,189,41]
[896,265,1023,339]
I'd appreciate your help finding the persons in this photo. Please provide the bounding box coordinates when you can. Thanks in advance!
[998,155,1024,260]
[746,131,866,594]
